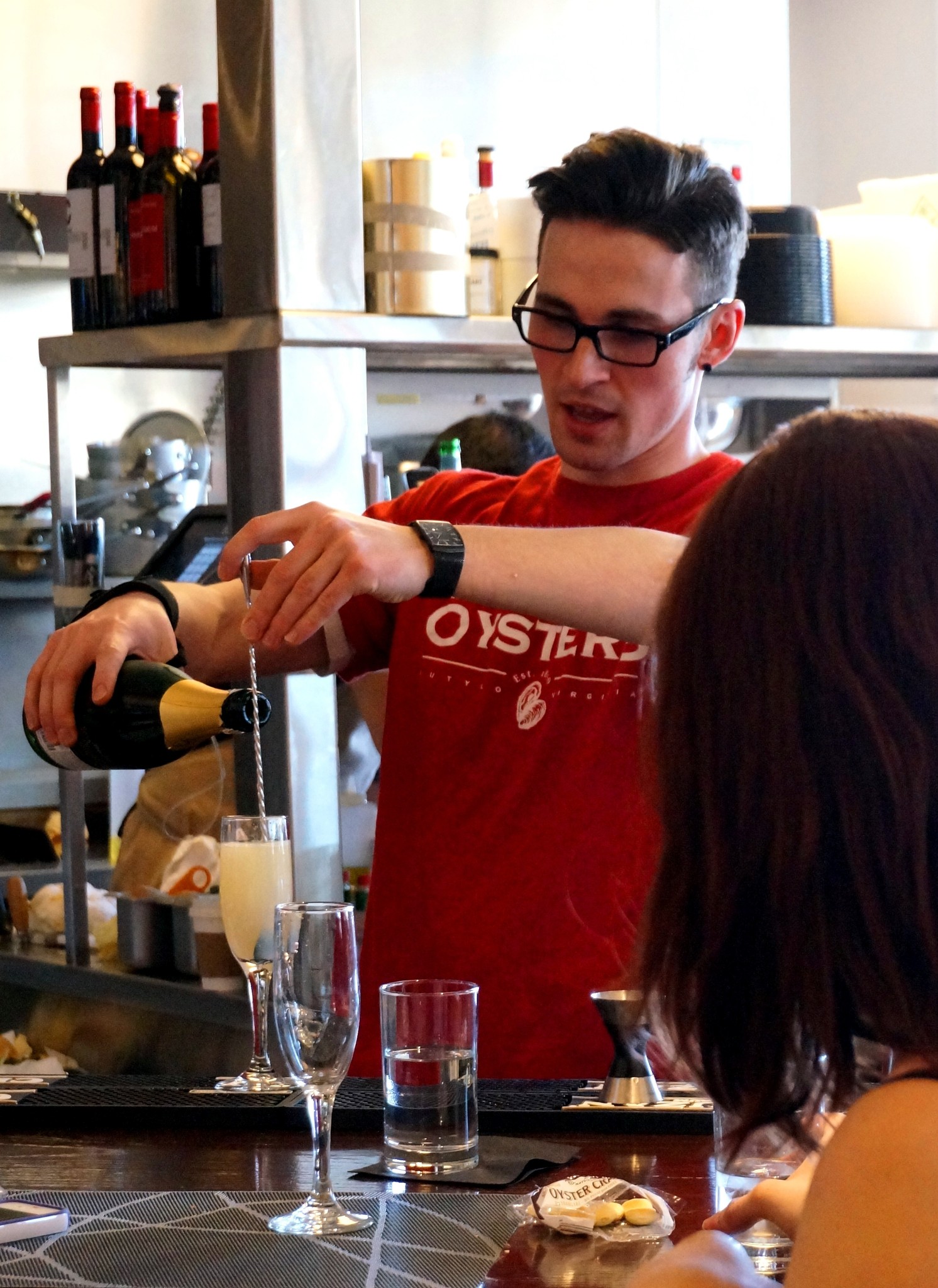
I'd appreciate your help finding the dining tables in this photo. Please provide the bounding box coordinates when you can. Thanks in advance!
[0,1077,719,1288]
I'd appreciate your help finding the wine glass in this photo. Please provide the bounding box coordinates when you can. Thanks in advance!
[216,814,296,1095]
[269,899,374,1236]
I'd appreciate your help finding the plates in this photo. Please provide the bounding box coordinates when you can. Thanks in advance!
[120,408,211,483]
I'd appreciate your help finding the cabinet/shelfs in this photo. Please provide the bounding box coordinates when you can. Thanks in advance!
[40,309,938,1012]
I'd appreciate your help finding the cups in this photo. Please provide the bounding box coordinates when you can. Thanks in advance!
[379,979,482,1176]
[190,893,246,992]
[712,1102,826,1276]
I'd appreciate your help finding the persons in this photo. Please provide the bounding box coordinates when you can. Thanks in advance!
[614,410,938,1286]
[21,127,751,1079]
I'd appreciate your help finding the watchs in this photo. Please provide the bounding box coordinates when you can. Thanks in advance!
[407,515,464,601]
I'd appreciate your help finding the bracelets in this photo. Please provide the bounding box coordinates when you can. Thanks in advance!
[80,579,179,630]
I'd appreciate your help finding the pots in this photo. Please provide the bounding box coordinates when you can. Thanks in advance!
[0,439,214,577]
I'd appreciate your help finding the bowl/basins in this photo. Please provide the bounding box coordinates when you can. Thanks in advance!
[734,204,835,327]
[116,893,198,977]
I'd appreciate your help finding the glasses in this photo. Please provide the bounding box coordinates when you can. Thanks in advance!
[512,273,734,367]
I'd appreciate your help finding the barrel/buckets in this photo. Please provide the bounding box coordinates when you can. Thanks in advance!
[363,153,472,318]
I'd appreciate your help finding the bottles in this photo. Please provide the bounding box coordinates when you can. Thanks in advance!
[438,437,462,473]
[127,82,223,327]
[67,86,108,335]
[98,81,145,331]
[468,142,505,317]
[22,660,273,772]
